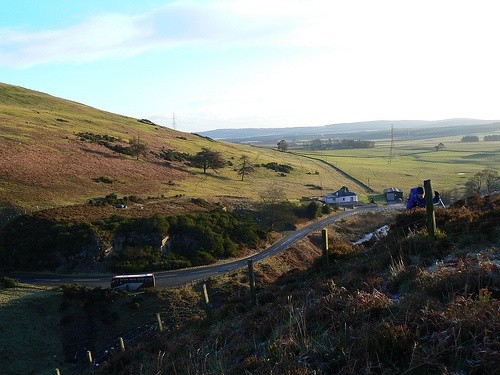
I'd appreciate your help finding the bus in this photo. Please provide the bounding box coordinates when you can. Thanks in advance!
[109,273,156,293]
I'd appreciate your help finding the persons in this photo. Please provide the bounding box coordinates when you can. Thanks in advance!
[406,187,441,210]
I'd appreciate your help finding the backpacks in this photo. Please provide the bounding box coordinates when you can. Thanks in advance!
[408,188,418,208]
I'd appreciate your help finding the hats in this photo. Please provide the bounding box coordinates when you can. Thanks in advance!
[417,187,425,194]
